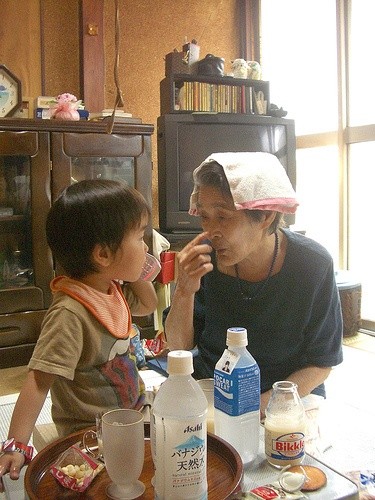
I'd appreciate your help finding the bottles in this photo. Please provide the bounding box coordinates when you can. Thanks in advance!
[264,381,307,470]
[152,349,209,500]
[213,326,261,466]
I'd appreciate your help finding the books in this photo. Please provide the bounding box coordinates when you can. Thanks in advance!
[175,81,268,114]
[90,109,143,125]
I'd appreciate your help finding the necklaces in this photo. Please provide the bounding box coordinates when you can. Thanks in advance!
[234,231,279,301]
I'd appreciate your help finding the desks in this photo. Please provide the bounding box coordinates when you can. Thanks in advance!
[152,212,307,356]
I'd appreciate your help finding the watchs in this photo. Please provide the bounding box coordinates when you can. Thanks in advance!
[1,438,33,466]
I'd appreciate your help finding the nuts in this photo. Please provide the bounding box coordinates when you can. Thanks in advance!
[60,464,102,487]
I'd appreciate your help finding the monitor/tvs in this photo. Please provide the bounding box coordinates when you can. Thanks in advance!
[158,114,297,234]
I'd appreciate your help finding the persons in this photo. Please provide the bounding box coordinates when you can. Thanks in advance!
[0,177,158,494]
[148,151,343,420]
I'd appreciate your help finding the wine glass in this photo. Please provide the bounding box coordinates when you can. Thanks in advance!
[101,408,146,500]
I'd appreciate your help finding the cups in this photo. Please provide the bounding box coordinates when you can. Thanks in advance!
[196,377,214,434]
[82,409,110,462]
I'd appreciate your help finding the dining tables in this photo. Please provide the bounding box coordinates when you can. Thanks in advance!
[3,399,361,500]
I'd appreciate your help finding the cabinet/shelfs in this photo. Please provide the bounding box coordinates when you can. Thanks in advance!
[0,115,155,368]
[158,74,272,116]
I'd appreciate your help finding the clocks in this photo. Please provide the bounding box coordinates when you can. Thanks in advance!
[0,64,23,117]
